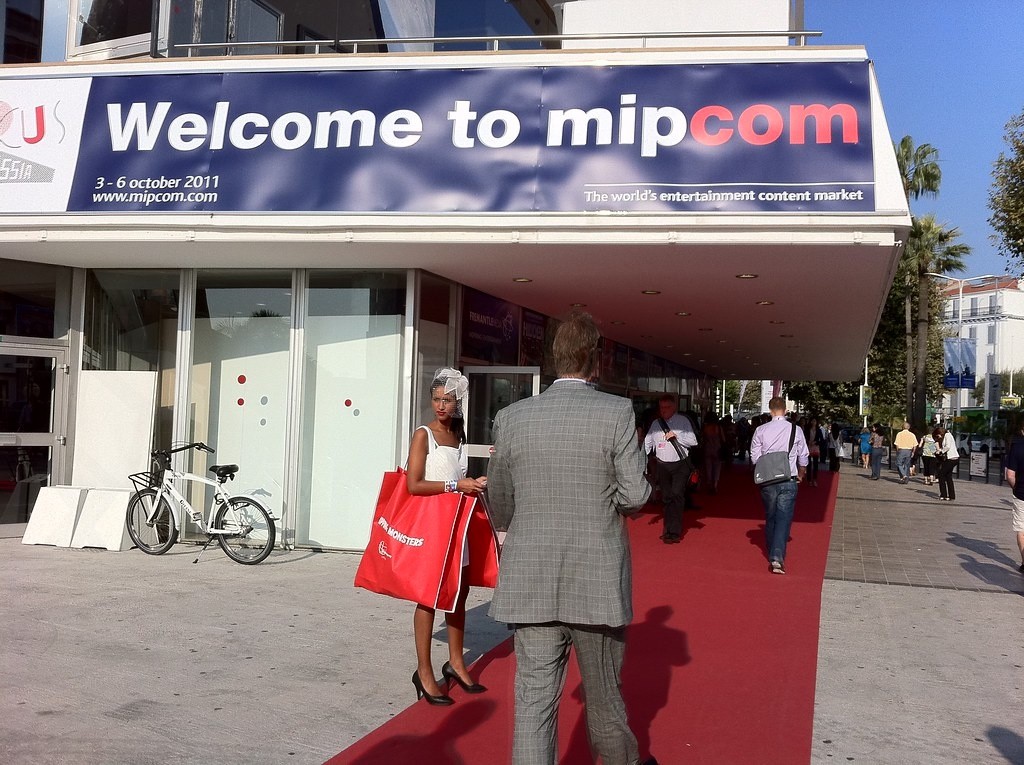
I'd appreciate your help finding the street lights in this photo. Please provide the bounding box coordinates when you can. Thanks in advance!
[926,271,995,475]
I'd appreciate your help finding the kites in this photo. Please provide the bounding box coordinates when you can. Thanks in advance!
[959,338,975,389]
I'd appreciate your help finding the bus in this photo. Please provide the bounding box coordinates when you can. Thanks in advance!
[952,409,1013,459]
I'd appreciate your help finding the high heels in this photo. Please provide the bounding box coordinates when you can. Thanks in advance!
[442,661,488,694]
[411,670,453,706]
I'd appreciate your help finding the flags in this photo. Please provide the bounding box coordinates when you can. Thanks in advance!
[989,374,1001,411]
[943,337,959,388]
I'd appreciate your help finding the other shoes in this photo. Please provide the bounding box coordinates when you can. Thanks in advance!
[707,486,719,495]
[923,475,934,486]
[721,451,751,472]
[862,461,869,468]
[868,475,880,480]
[819,461,826,465]
[909,464,916,476]
[772,561,786,573]
[658,531,680,543]
[898,475,909,484]
[933,476,939,483]
[828,470,839,474]
[807,479,818,486]
[936,495,956,501]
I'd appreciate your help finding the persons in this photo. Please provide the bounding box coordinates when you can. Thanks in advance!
[828,422,842,473]
[486,317,656,765]
[909,428,922,476]
[404,367,488,705]
[797,418,810,476]
[644,393,698,544]
[893,423,919,484]
[816,422,828,465]
[932,452,946,483]
[698,410,726,494]
[803,418,824,487]
[1005,421,1024,576]
[634,423,645,449]
[682,409,701,512]
[869,423,883,481]
[826,425,832,462]
[919,425,936,485]
[721,412,798,477]
[857,428,872,469]
[932,425,960,501]
[749,395,810,573]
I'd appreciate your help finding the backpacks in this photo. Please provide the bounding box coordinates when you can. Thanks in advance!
[753,451,791,486]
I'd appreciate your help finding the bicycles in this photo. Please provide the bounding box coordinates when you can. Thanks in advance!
[126,441,279,564]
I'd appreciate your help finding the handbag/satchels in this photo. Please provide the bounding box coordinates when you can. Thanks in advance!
[806,441,820,456]
[835,446,845,458]
[467,490,503,589]
[935,451,947,462]
[685,460,704,491]
[916,436,925,456]
[351,425,477,613]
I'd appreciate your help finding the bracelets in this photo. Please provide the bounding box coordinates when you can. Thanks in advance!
[444,480,458,493]
